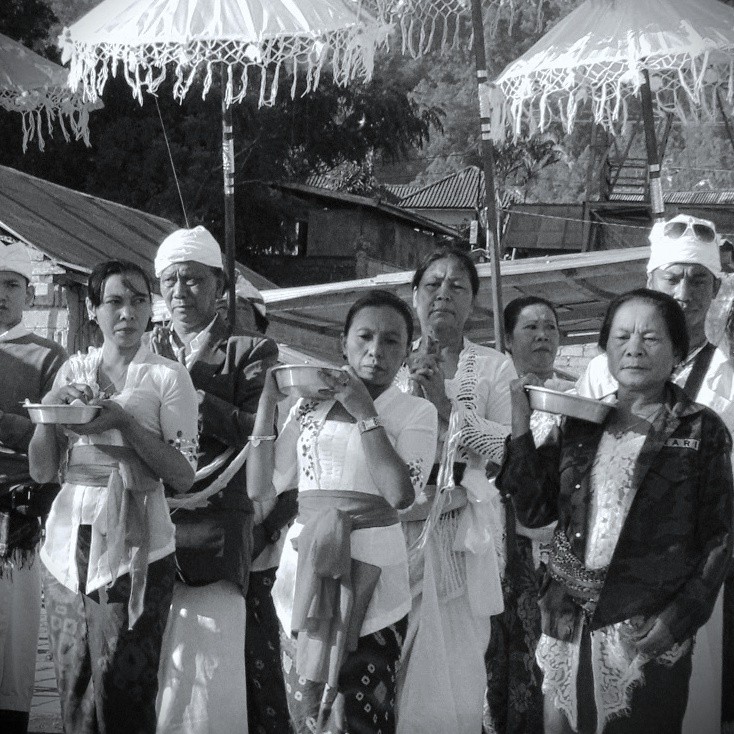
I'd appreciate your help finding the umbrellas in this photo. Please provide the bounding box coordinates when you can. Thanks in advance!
[0,33,105,154]
[57,0,387,325]
[488,0,734,216]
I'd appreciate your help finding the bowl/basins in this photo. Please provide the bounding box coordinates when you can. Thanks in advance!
[271,365,349,400]
[22,403,104,425]
[524,385,617,424]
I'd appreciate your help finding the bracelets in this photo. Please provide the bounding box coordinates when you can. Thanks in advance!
[246,435,277,447]
[356,416,384,435]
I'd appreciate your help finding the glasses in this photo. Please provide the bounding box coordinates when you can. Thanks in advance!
[658,222,718,247]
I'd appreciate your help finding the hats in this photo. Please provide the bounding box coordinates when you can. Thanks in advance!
[647,214,722,279]
[0,241,33,282]
[154,226,223,278]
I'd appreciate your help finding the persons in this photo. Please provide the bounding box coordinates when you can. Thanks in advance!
[133,226,278,734]
[504,286,734,734]
[28,260,199,734]
[502,294,574,446]
[0,238,104,734]
[386,248,513,734]
[534,212,734,734]
[234,275,269,338]
[245,291,438,734]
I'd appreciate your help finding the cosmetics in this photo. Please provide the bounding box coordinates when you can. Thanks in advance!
[349,0,582,350]
